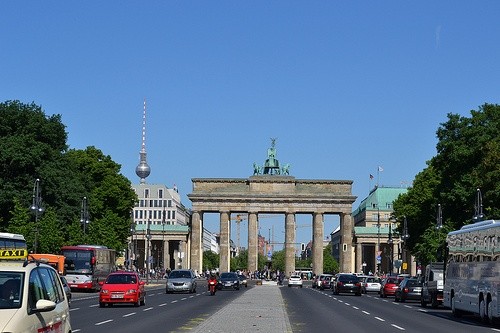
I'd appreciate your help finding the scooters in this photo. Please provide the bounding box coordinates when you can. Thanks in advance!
[208,279,217,296]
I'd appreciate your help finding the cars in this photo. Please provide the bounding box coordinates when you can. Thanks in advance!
[312,272,366,291]
[99,271,146,308]
[218,272,240,291]
[395,278,422,303]
[239,275,247,288]
[288,275,303,288]
[59,276,72,308]
[333,273,362,296]
[381,277,402,298]
[362,277,382,294]
[397,274,411,284]
[0,248,72,333]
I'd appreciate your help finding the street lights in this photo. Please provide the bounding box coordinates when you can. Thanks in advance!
[471,188,483,223]
[129,222,136,270]
[386,222,394,276]
[79,197,89,244]
[401,215,409,274]
[436,203,444,244]
[30,178,43,254]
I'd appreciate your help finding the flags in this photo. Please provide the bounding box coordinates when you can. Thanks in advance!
[370,174,374,180]
[378,167,383,172]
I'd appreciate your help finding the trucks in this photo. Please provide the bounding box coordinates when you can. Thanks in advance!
[27,254,65,274]
[421,261,444,310]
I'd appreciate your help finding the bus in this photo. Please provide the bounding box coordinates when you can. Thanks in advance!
[59,245,118,293]
[436,220,500,327]
[0,233,29,254]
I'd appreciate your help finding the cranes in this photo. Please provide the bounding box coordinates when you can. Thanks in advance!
[230,213,282,257]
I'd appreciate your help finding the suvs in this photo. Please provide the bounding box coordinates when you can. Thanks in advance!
[164,268,197,294]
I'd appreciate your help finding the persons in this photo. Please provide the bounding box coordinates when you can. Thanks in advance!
[238,269,252,279]
[166,268,171,277]
[255,267,285,284]
[210,269,216,274]
[298,271,314,280]
[206,269,210,278]
[368,270,386,281]
[207,272,219,291]
